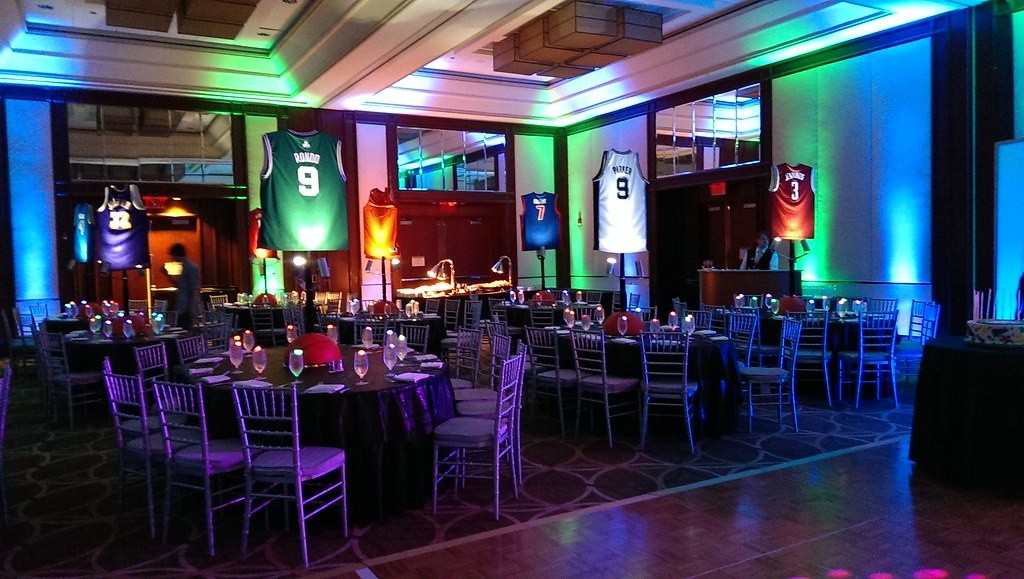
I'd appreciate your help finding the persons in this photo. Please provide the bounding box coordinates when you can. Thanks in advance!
[739,230,780,272]
[159,242,204,335]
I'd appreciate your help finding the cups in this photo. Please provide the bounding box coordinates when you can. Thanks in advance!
[328,328,338,342]
[287,329,297,344]
[386,333,399,347]
[362,328,373,354]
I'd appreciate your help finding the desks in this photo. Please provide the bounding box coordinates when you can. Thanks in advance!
[399,290,537,327]
[495,301,602,344]
[64,325,193,373]
[908,337,1024,491]
[187,345,455,445]
[721,304,901,353]
[42,314,117,332]
[537,324,741,440]
[340,312,447,354]
[222,301,286,330]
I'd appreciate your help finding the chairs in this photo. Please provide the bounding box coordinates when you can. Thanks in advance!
[0,292,941,568]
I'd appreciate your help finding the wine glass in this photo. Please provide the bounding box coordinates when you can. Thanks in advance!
[243,332,254,357]
[395,338,407,367]
[229,346,244,374]
[62,300,165,340]
[563,306,698,340]
[354,351,370,385]
[289,352,304,384]
[383,345,397,377]
[252,349,267,379]
[734,293,868,321]
[509,289,582,309]
[349,299,420,320]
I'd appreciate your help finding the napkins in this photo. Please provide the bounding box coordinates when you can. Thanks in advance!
[351,344,380,350]
[188,368,215,375]
[202,375,231,384]
[412,354,438,362]
[651,340,681,346]
[196,357,223,364]
[694,330,717,336]
[303,384,344,393]
[581,334,601,340]
[660,324,679,330]
[710,336,729,341]
[233,379,273,388]
[544,325,561,330]
[576,322,594,327]
[394,372,430,383]
[420,362,444,369]
[222,350,250,357]
[554,330,571,334]
[611,337,637,344]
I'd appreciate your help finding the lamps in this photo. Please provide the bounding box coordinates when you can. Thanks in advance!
[491,255,512,284]
[605,257,617,276]
[427,259,455,289]
